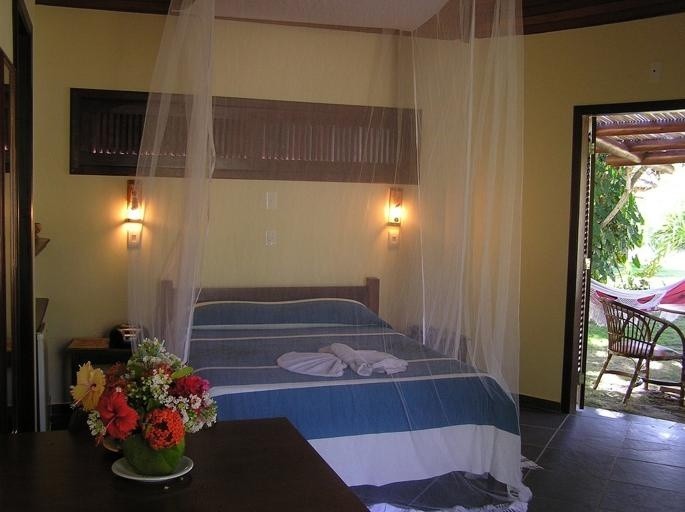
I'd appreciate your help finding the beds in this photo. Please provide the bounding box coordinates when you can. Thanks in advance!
[158,276,519,510]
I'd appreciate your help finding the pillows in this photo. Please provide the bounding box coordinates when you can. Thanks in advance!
[170,298,395,329]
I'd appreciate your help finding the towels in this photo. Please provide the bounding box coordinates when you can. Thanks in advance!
[276,341,408,378]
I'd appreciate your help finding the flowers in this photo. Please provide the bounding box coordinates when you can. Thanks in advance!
[68,337,219,451]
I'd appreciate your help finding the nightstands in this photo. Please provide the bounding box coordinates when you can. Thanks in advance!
[64,338,135,389]
[409,325,467,363]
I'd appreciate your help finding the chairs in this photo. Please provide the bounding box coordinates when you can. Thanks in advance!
[592,295,685,409]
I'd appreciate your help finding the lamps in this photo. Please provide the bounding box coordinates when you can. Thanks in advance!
[123,181,144,248]
[388,187,403,248]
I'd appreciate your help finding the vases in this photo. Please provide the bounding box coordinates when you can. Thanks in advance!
[124,431,186,475]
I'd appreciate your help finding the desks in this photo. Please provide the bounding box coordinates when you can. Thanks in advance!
[1,417,372,511]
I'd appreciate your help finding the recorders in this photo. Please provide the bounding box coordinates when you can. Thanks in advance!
[109,324,149,347]
